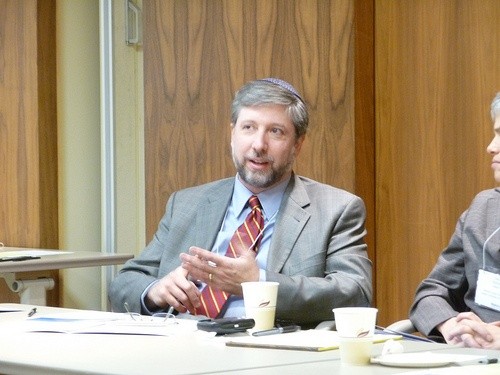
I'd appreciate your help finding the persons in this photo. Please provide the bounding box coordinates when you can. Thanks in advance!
[108,78,374,331]
[408,91,500,349]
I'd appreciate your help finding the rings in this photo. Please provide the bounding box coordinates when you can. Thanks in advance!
[208,273,213,283]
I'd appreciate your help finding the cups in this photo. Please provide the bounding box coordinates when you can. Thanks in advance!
[332,307,378,368]
[241,281,280,334]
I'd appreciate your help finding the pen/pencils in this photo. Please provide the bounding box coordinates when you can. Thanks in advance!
[252,325,301,336]
[478,358,498,363]
[28,308,36,316]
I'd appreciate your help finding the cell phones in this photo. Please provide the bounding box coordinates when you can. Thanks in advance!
[198,316,254,332]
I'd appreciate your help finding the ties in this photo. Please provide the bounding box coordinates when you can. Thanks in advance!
[195,195,264,317]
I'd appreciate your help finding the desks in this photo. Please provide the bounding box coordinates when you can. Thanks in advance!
[0,302,500,375]
[0,247,135,306]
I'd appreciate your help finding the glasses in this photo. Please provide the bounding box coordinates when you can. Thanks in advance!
[122,302,179,327]
